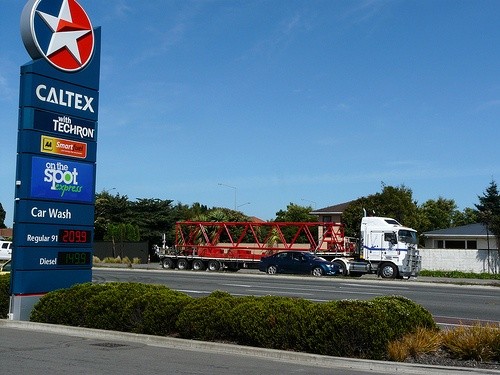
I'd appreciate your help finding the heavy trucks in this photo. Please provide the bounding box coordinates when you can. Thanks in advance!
[152,205,422,280]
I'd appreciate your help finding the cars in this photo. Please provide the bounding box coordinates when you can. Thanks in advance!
[0,240,12,261]
[258,250,344,277]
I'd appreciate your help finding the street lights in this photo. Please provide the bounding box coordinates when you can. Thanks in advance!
[218,183,236,210]
